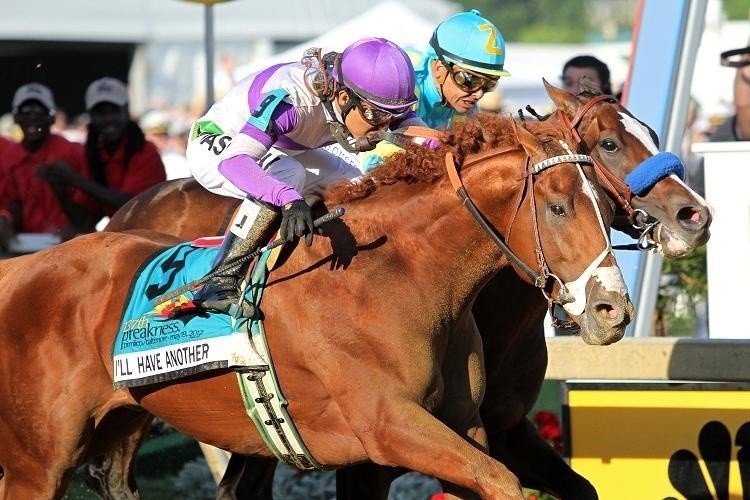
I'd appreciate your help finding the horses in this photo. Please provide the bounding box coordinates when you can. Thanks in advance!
[99,69,713,499]
[1,112,640,499]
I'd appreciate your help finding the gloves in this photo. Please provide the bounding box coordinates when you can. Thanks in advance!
[281,199,323,247]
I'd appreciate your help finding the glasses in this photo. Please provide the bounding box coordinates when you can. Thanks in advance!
[19,105,46,115]
[446,63,500,92]
[345,91,412,120]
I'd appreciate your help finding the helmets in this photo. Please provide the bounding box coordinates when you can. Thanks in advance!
[334,38,419,118]
[430,10,512,79]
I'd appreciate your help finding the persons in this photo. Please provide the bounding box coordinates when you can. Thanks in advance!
[413,9,509,128]
[37,77,166,224]
[188,37,449,319]
[613,85,616,88]
[560,57,611,102]
[0,82,84,248]
[0,137,21,250]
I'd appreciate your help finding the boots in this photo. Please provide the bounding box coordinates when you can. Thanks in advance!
[196,194,277,317]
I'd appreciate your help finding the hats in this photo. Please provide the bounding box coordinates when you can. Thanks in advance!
[85,77,130,110]
[15,84,58,114]
